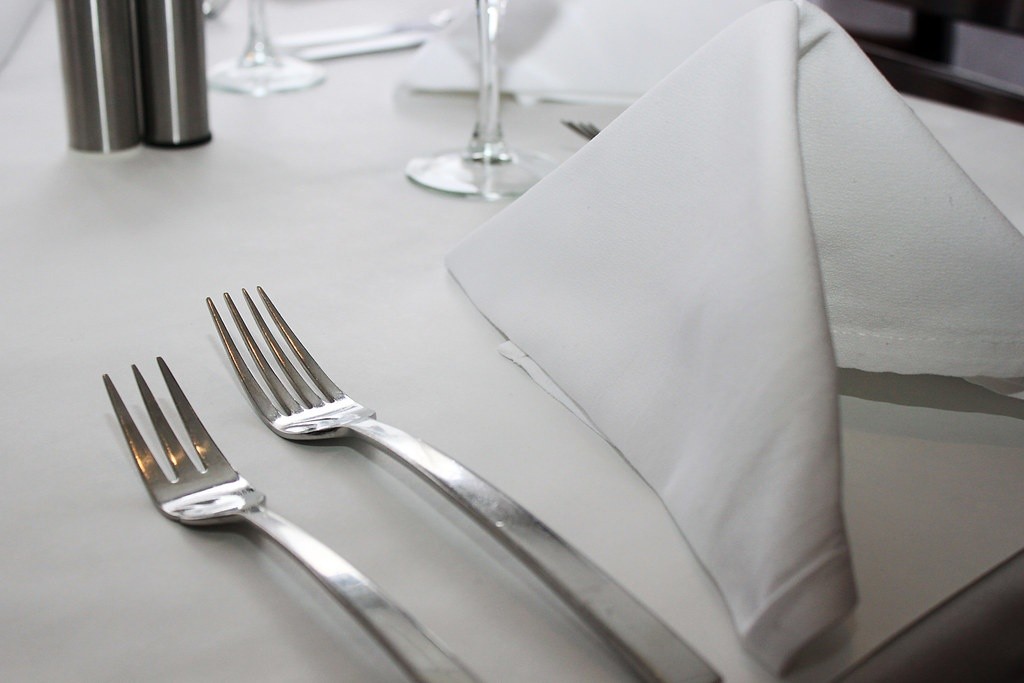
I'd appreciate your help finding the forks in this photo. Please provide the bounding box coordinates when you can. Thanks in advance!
[205,284,723,683]
[96,355,478,683]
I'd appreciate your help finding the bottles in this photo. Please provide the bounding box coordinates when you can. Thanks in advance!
[138,0,212,151]
[51,1,143,156]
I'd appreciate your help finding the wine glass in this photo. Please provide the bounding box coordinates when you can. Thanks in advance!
[207,0,330,97]
[402,0,560,198]
